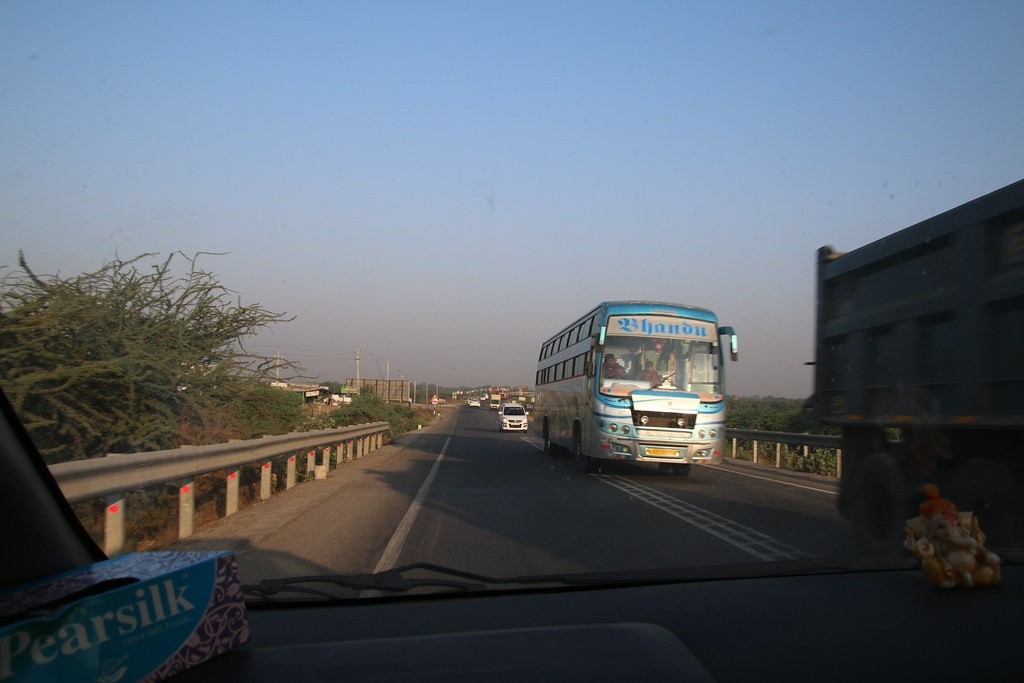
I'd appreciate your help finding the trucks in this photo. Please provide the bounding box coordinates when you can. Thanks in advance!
[798,179,1023,553]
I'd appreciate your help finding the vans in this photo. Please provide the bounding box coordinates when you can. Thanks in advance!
[497,402,529,433]
[466,392,507,410]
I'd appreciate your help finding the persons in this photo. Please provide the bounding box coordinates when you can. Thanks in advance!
[602,353,627,379]
[635,360,659,389]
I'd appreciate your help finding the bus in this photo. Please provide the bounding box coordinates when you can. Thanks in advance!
[534,301,739,472]
